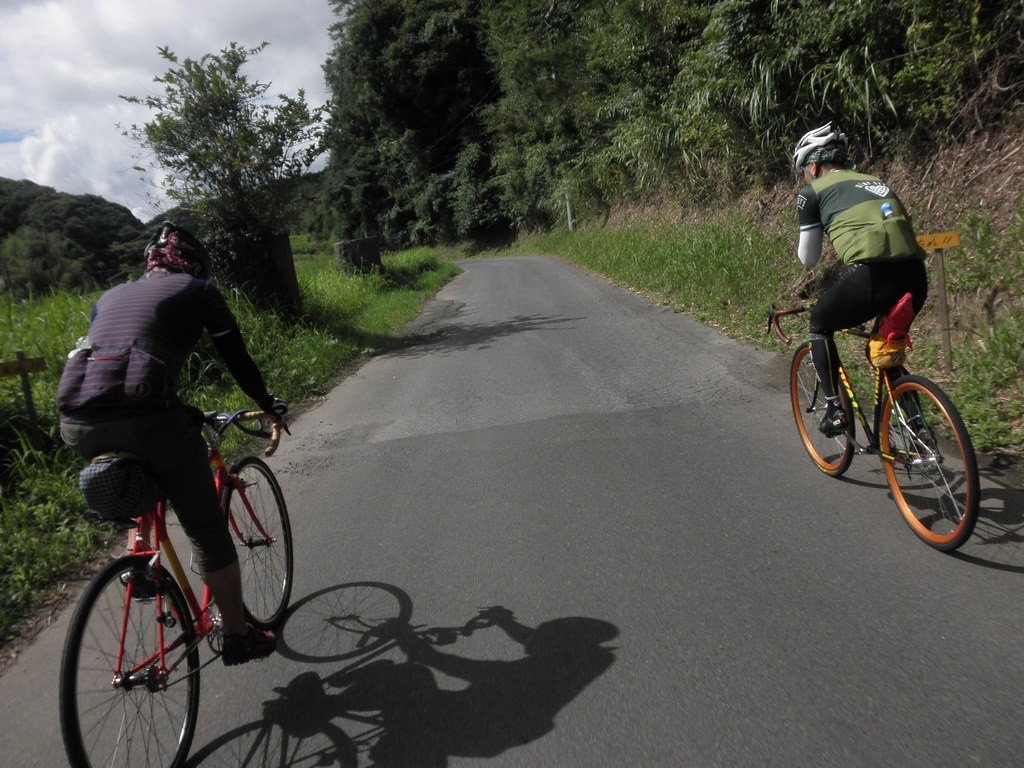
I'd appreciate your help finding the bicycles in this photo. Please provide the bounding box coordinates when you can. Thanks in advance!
[760,302,980,553]
[58,407,296,768]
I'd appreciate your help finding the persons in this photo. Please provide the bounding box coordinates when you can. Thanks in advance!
[54,222,294,670]
[790,120,946,472]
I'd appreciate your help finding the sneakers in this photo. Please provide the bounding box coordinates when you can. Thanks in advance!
[818,405,848,435]
[908,425,933,460]
[222,618,280,665]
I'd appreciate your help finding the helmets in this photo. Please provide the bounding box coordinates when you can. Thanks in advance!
[144,221,210,279]
[790,121,849,171]
